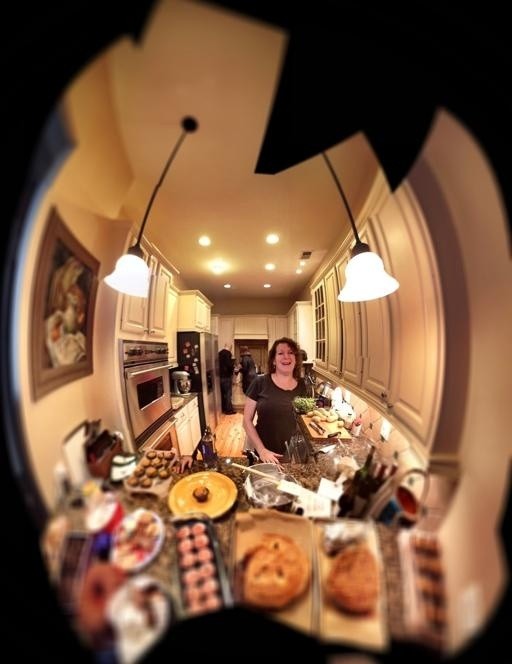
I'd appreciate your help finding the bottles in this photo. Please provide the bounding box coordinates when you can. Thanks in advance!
[338,445,397,512]
[80,480,110,526]
[344,414,354,428]
[201,430,214,464]
[321,381,331,398]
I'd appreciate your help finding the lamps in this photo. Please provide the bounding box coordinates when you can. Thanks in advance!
[320,149,400,304]
[101,117,198,299]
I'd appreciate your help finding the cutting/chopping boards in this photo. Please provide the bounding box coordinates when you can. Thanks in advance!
[300,413,352,443]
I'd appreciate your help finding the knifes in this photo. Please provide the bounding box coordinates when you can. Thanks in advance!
[328,429,350,438]
[312,444,337,458]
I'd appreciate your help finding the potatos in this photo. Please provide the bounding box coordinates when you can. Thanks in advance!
[307,407,345,427]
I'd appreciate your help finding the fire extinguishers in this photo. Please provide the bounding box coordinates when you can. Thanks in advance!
[201,427,216,460]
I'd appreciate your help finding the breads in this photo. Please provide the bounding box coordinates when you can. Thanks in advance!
[326,547,379,612]
[234,534,312,608]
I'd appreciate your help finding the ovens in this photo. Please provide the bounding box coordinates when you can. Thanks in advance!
[120,339,180,456]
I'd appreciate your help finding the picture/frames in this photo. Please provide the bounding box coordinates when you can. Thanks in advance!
[28,203,102,404]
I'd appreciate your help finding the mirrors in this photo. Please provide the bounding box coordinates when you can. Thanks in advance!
[1,1,511,663]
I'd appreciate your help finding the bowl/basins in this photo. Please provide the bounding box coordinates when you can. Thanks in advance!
[241,461,298,512]
[86,501,125,536]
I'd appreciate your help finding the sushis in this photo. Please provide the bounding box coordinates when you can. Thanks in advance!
[129,452,174,488]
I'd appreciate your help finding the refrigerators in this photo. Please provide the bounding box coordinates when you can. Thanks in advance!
[177,331,222,432]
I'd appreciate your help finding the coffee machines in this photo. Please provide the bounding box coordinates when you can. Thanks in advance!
[172,370,191,396]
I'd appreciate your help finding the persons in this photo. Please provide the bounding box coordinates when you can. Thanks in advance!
[218,337,309,467]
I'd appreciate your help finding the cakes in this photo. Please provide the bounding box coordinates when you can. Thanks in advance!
[194,487,209,502]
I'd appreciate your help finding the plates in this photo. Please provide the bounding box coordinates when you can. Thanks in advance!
[101,575,172,664]
[167,471,238,521]
[107,511,166,573]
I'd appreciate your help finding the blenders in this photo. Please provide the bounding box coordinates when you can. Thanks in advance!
[301,363,316,397]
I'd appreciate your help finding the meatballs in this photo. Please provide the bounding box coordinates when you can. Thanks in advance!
[178,523,220,612]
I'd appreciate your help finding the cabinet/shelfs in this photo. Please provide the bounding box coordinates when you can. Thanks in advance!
[283,166,471,477]
[106,219,214,462]
